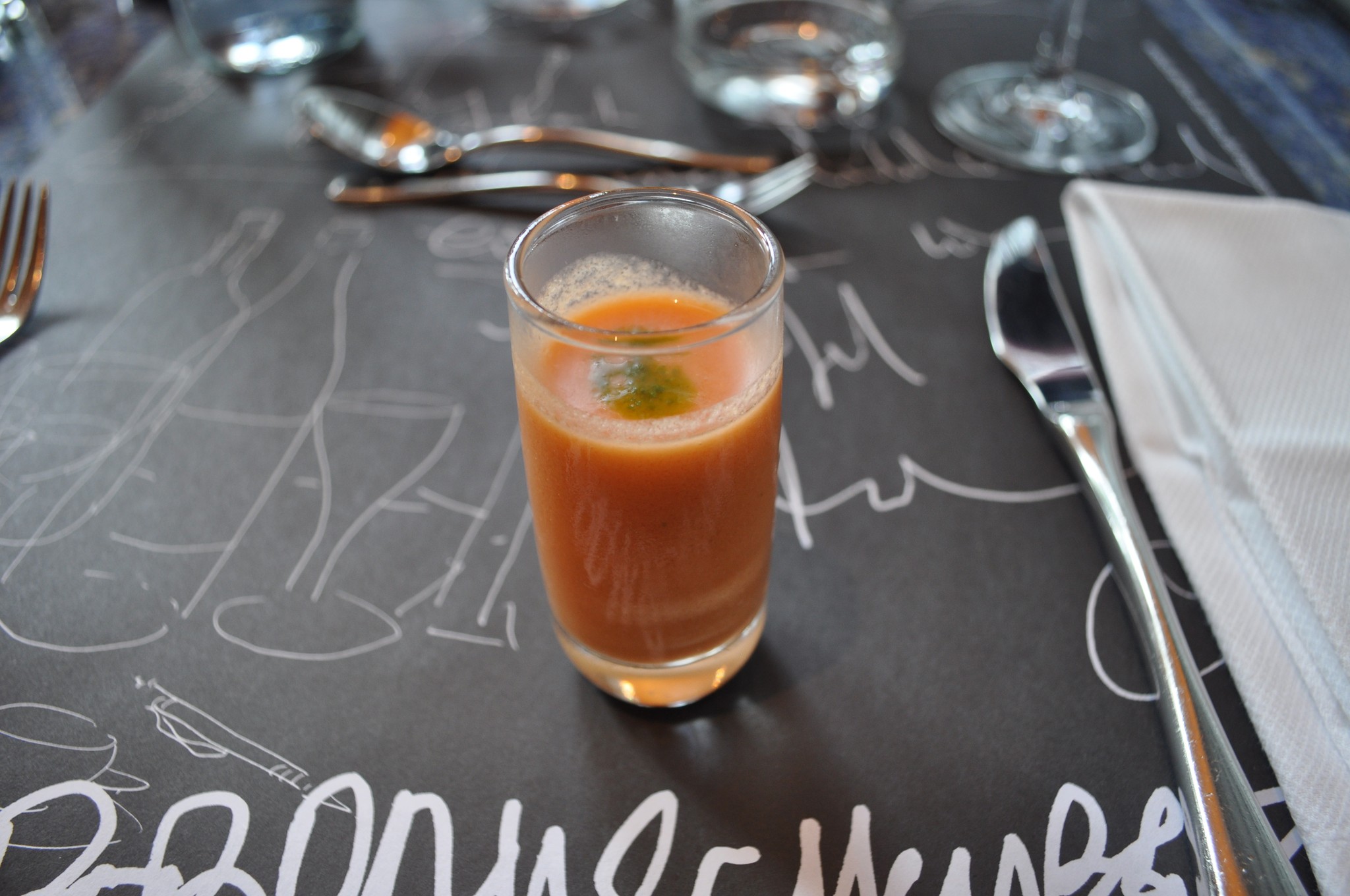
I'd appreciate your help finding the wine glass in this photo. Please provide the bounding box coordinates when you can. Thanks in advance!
[927,0,1159,175]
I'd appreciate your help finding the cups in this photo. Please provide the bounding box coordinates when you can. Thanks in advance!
[169,0,369,78]
[673,0,904,129]
[504,189,786,708]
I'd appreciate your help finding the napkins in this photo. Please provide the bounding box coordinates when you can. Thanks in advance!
[1058,170,1350,896]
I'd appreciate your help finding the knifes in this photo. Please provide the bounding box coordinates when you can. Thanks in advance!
[982,213,1250,896]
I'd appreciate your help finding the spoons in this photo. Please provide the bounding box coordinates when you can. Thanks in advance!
[290,85,780,175]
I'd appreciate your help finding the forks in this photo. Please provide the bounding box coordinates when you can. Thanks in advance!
[0,175,48,344]
[325,152,821,217]
